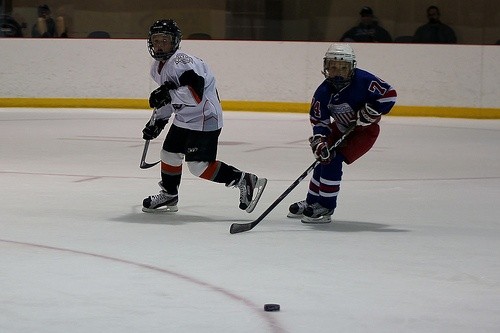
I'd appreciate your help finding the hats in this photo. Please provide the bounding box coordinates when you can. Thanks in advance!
[147,19,183,62]
[360,7,373,17]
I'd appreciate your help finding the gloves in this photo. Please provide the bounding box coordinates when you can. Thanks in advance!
[309,134,336,165]
[142,119,169,140]
[149,86,172,109]
[349,103,382,127]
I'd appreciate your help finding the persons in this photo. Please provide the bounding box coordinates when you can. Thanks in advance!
[339,7,392,42]
[0,0,24,38]
[414,6,457,44]
[287,44,398,224]
[142,19,268,213]
[31,5,67,38]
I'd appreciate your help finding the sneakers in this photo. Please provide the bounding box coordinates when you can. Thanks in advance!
[239,173,267,213]
[141,191,179,213]
[287,200,310,217]
[301,202,335,223]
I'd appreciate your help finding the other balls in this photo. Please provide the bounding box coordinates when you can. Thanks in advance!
[264,304,280,311]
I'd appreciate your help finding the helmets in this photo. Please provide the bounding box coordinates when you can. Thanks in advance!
[323,42,356,71]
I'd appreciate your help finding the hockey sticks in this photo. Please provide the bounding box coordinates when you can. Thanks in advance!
[140,107,161,169]
[230,122,357,234]
